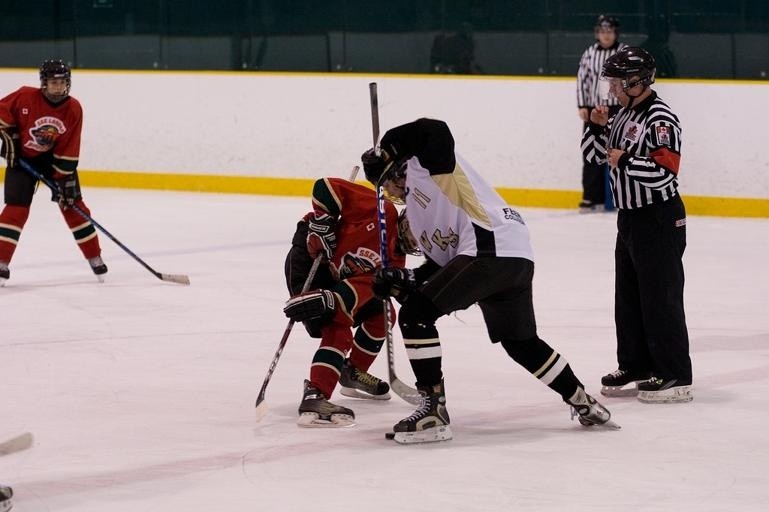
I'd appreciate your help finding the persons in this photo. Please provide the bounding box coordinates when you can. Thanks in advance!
[360,117,611,430]
[0,58,108,277]
[284,177,421,420]
[580,45,693,392]
[576,15,626,208]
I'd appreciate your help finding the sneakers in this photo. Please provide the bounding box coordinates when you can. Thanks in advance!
[577,196,607,211]
[600,362,695,394]
[340,354,391,396]
[297,378,355,422]
[0,261,11,280]
[85,254,108,274]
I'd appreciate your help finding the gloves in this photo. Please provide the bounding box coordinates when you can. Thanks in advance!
[2,130,20,166]
[359,138,403,188]
[369,264,421,307]
[282,285,338,326]
[56,177,78,206]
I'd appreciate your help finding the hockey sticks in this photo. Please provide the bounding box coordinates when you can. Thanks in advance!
[256,163,359,424]
[0,433,31,456]
[370,83,427,405]
[18,156,190,284]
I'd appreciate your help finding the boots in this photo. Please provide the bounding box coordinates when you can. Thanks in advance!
[565,385,613,428]
[392,377,452,432]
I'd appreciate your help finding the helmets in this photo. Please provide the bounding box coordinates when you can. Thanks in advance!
[593,13,622,47]
[599,46,658,100]
[38,58,72,104]
